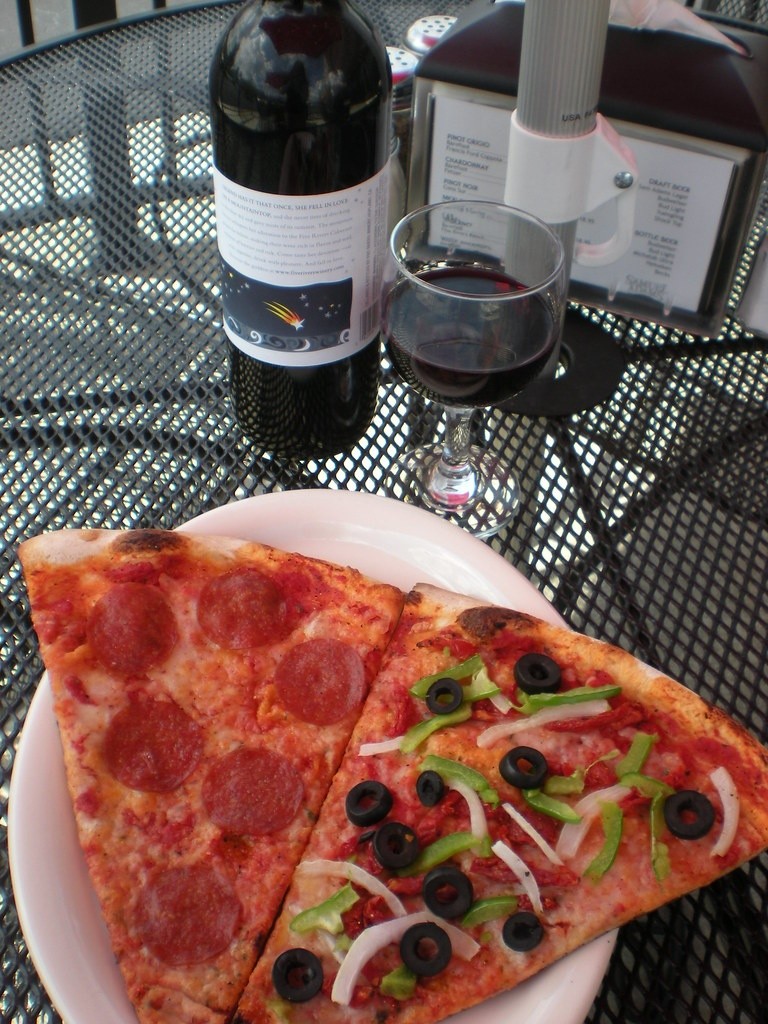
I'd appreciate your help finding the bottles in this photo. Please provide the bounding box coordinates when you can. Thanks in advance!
[210,0,389,462]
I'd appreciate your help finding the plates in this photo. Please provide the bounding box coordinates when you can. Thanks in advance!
[8,488,619,1022]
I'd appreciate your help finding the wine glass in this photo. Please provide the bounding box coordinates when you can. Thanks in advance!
[378,199,568,545]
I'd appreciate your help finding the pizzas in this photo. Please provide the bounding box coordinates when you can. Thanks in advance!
[226,583,768,1024]
[17,522,408,1023]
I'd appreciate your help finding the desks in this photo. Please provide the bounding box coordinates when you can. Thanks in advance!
[0,0,768,1024]
